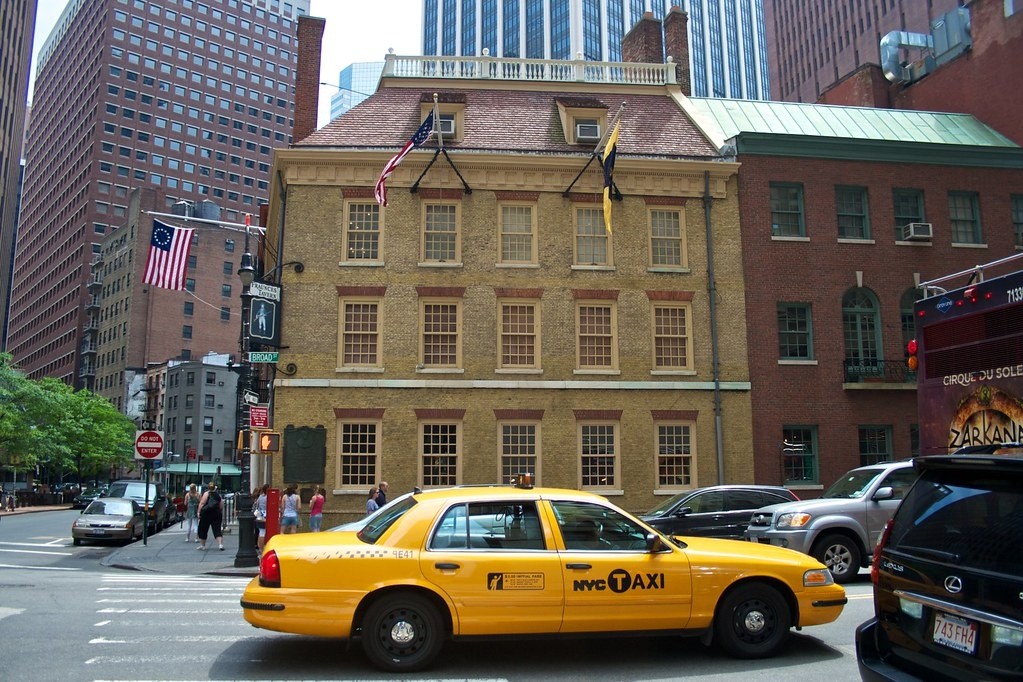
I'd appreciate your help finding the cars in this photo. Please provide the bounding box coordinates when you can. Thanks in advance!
[73,480,184,545]
[32,479,75,494]
[241,471,848,671]
[638,483,803,542]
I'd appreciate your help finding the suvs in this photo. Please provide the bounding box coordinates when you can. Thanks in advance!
[745,458,918,583]
[856,442,1023,682]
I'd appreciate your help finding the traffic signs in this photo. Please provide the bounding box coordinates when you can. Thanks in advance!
[243,390,259,407]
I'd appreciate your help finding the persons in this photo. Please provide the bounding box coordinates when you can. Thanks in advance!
[196,482,225,551]
[571,520,599,549]
[375,481,389,508]
[184,483,201,543]
[280,483,301,534]
[365,488,379,515]
[251,484,270,547]
[309,484,324,531]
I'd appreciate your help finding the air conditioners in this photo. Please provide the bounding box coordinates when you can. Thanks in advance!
[576,123,601,143]
[434,118,456,139]
[900,222,933,242]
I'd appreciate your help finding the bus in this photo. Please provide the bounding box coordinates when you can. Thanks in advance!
[906,250,1023,455]
[0,465,42,493]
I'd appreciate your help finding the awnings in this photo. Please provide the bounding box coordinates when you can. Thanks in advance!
[156,464,241,476]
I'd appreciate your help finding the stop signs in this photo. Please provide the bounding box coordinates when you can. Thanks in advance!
[135,430,164,459]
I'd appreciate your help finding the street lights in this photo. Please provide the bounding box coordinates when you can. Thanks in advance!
[166,451,180,493]
[233,227,259,568]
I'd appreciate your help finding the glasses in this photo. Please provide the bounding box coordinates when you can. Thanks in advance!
[375,492,379,494]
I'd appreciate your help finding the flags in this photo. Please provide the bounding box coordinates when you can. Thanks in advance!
[141,220,195,291]
[602,119,620,236]
[374,108,435,207]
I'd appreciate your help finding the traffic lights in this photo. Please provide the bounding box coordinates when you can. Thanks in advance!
[261,433,280,453]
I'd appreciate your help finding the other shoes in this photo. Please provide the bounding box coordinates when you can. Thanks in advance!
[196,545,206,551]
[185,538,189,542]
[217,545,225,551]
[194,538,199,542]
